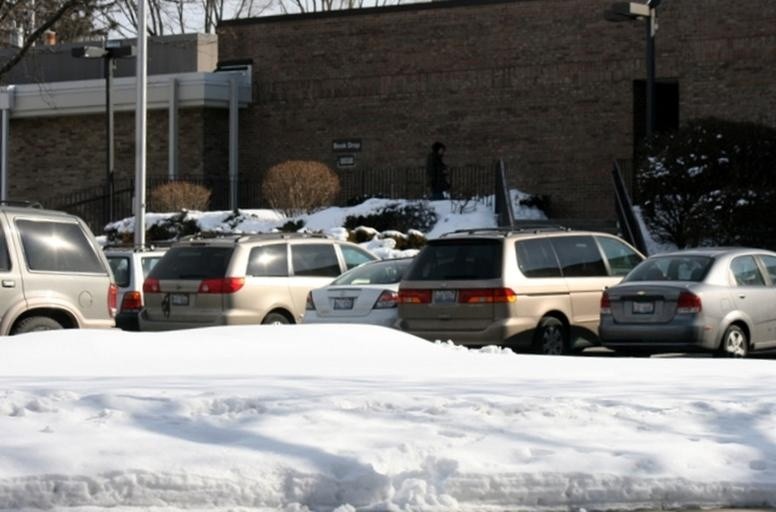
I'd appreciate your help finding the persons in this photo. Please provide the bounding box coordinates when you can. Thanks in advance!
[425,141,449,201]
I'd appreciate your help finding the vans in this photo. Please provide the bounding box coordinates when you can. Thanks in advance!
[103,238,381,331]
[0,205,117,340]
[398,223,646,353]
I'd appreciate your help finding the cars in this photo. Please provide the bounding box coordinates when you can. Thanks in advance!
[304,254,413,331]
[599,245,776,357]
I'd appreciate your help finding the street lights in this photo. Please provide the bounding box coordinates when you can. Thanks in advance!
[71,43,136,220]
[603,0,660,149]
